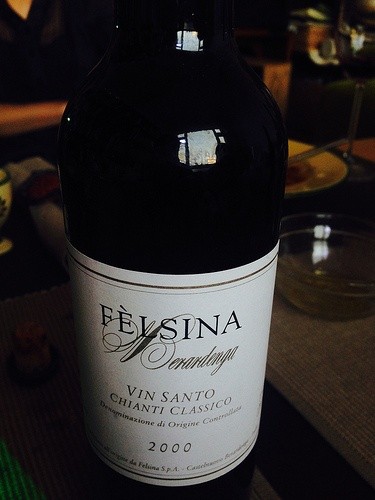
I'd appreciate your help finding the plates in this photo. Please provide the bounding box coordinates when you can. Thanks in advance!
[283,140,349,199]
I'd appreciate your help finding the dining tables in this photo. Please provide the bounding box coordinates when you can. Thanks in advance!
[0,122,375,500]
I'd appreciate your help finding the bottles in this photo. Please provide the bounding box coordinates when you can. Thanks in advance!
[58,0,287,500]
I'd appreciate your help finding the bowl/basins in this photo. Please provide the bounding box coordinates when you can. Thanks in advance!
[276,214,375,320]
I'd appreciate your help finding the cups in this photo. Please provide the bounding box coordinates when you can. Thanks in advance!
[0,168,14,257]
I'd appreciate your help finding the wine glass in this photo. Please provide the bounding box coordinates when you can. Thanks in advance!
[334,0,375,176]
